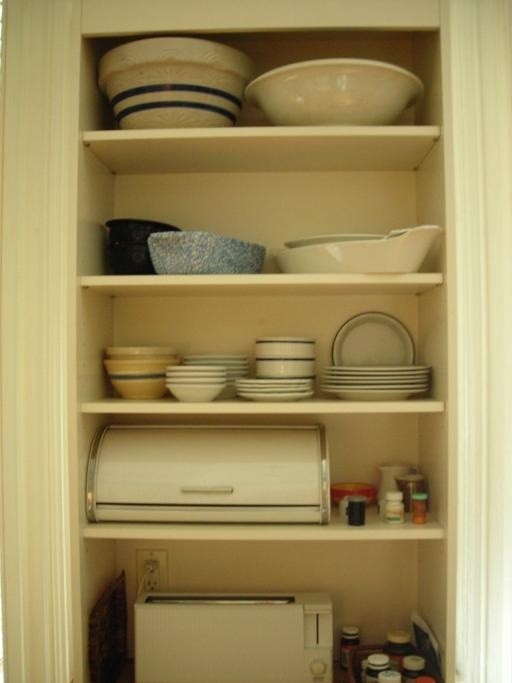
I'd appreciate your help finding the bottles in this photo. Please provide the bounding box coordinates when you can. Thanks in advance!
[377,670,402,683]
[383,492,405,524]
[340,626,361,668]
[411,492,429,525]
[402,656,427,683]
[363,654,390,683]
[348,495,366,526]
[381,628,417,672]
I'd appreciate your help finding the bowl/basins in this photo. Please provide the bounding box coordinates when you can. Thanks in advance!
[104,219,182,272]
[332,483,376,505]
[272,224,444,275]
[165,366,227,401]
[243,57,426,126]
[146,232,266,274]
[105,345,181,398]
[254,338,315,379]
[96,37,254,129]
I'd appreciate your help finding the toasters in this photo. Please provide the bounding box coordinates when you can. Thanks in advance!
[132,591,333,683]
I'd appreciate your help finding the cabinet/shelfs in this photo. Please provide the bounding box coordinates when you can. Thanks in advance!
[68,0,457,683]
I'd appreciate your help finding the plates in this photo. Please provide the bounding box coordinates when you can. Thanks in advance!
[183,355,249,397]
[318,364,432,403]
[333,310,414,364]
[234,376,315,402]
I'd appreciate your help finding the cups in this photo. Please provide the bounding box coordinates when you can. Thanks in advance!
[394,475,425,509]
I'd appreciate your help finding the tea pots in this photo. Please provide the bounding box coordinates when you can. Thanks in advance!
[378,462,421,505]
